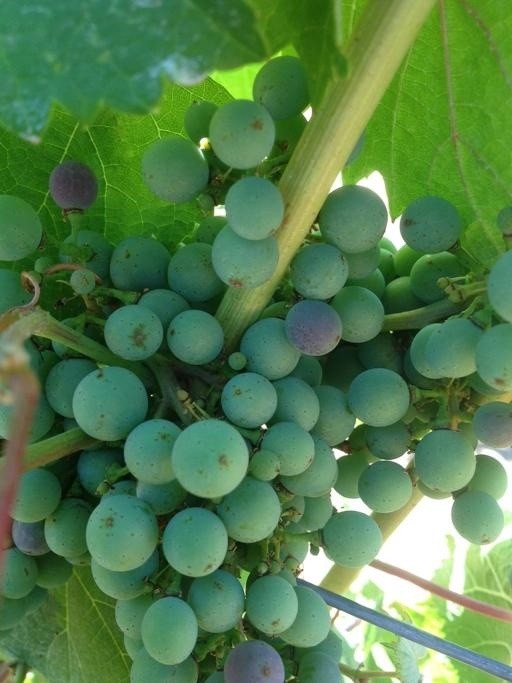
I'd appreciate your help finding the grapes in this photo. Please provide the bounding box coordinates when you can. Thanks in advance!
[1,56,512,683]
